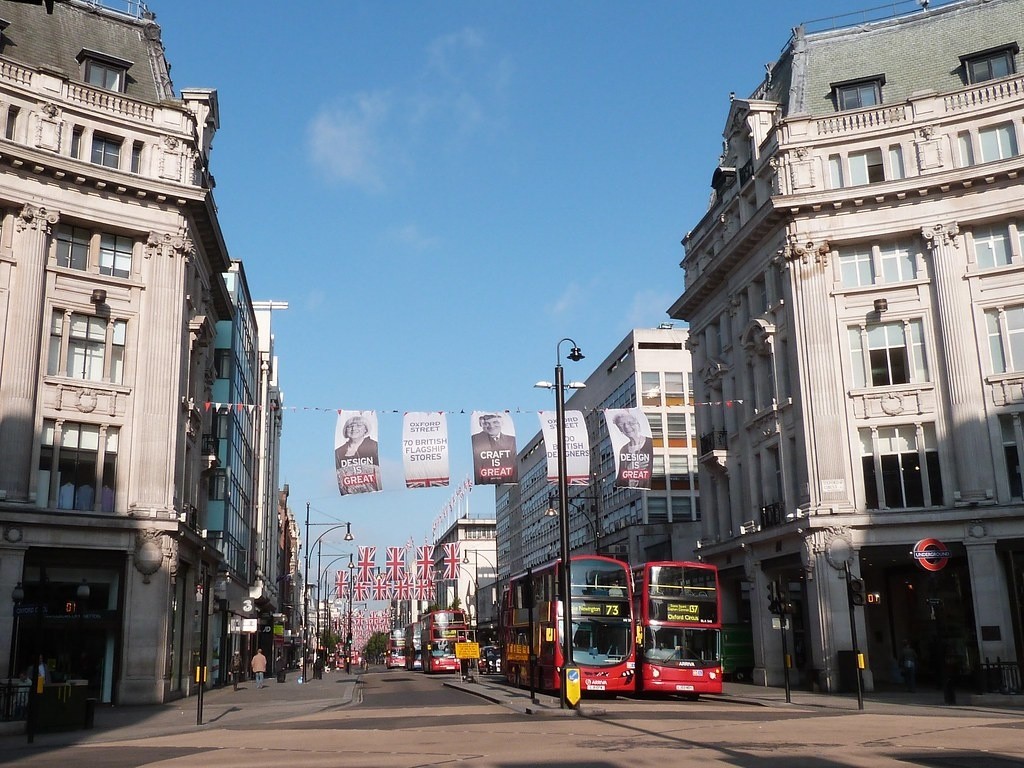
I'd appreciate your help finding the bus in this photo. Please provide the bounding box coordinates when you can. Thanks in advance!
[386,628,406,669]
[500,555,642,696]
[336,643,359,668]
[604,562,723,700]
[404,622,421,670]
[419,611,468,673]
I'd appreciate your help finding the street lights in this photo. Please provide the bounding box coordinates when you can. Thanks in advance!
[543,494,601,552]
[463,550,479,637]
[314,554,354,677]
[4,581,23,721]
[302,521,354,684]
[74,576,90,680]
[536,337,589,707]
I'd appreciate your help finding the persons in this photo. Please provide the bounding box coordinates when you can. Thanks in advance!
[228,651,245,691]
[609,412,652,491]
[251,649,267,689]
[14,673,32,706]
[276,655,287,683]
[471,414,518,485]
[897,641,917,693]
[335,418,382,494]
[315,655,324,679]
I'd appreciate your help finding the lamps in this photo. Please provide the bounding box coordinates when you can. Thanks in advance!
[874,299,888,314]
[11,581,24,618]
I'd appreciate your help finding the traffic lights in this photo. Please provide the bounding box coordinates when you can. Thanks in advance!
[851,579,880,606]
[777,591,791,612]
[347,632,352,644]
[209,588,220,616]
[768,581,778,613]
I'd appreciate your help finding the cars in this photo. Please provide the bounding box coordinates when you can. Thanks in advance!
[478,646,500,674]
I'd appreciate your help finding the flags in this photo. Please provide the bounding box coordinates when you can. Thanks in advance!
[333,480,472,653]
